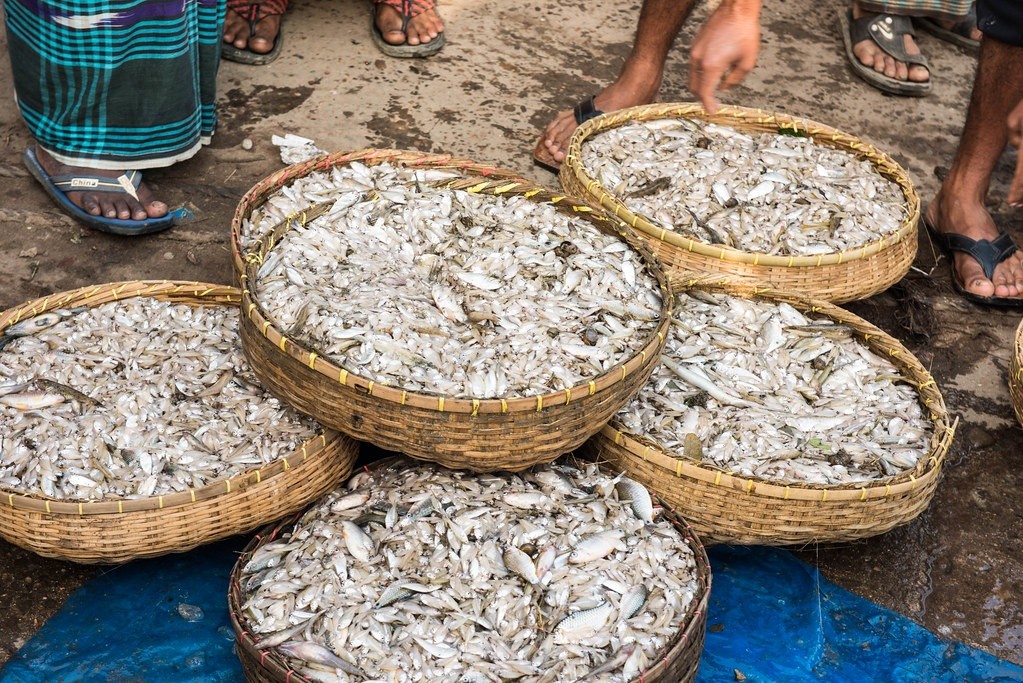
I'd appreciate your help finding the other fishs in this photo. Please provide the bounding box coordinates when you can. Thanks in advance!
[0,117,933,683]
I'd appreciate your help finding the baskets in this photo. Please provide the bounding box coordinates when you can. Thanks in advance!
[0,278,361,566]
[240,176,675,474]
[229,148,552,300]
[582,269,960,543]
[227,449,714,683]
[560,102,920,304]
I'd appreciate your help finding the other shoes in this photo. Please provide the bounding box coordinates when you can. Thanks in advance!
[985,199,1023,222]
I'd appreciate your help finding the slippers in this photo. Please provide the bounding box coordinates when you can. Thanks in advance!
[22,138,173,236]
[926,203,1023,309]
[534,95,604,174]
[838,5,932,96]
[370,0,445,57]
[921,5,984,52]
[219,0,290,65]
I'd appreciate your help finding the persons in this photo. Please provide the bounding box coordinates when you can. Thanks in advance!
[533,1,1022,308]
[833,1,986,100]
[221,0,448,67]
[3,0,228,237]
